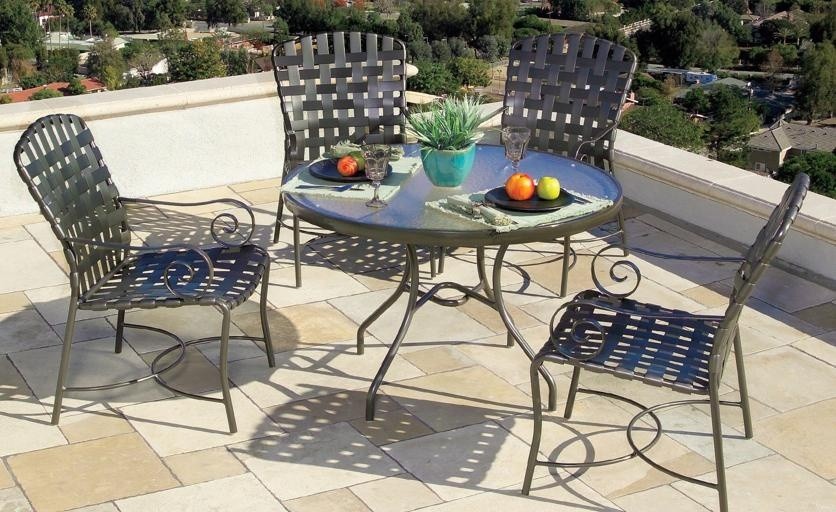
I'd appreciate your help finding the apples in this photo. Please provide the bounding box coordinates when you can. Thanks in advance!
[337,156,358,177]
[350,152,365,170]
[506,172,536,201]
[537,175,560,201]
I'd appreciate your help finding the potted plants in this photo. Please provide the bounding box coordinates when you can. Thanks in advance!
[398,86,514,189]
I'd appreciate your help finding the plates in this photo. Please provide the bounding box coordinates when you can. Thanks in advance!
[309,158,392,181]
[485,184,573,210]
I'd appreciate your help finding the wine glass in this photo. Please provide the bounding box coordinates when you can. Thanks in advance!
[361,143,391,208]
[502,126,531,174]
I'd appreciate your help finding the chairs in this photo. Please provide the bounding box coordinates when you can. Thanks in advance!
[439,28,639,299]
[267,27,436,289]
[522,170,811,512]
[9,108,278,435]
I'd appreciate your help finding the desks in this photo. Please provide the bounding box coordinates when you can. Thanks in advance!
[282,142,625,421]
